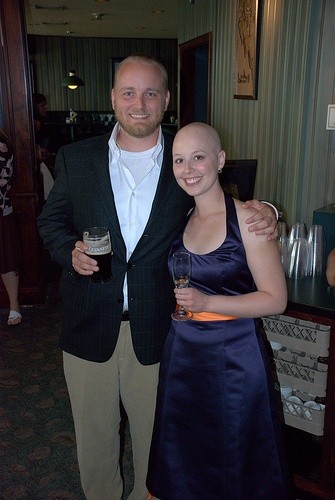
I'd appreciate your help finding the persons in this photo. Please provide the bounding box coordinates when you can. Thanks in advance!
[0,128,22,326]
[146,122,288,500]
[38,55,278,500]
[32,93,50,160]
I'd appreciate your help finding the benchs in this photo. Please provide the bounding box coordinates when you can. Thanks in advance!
[45,109,116,155]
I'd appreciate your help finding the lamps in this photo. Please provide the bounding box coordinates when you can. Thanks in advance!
[60,30,85,90]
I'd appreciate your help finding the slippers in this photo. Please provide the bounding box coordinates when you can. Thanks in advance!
[7,310,21,325]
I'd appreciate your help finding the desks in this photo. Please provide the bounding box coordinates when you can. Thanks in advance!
[255,278,335,500]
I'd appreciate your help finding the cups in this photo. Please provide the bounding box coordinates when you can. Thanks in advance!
[276,222,323,281]
[82,226,113,285]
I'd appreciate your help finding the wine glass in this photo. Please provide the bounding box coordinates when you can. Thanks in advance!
[171,252,192,321]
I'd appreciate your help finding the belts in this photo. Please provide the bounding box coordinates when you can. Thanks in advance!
[121,313,129,321]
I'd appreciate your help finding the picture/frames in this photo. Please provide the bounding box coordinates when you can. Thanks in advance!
[110,56,124,88]
[230,0,260,101]
